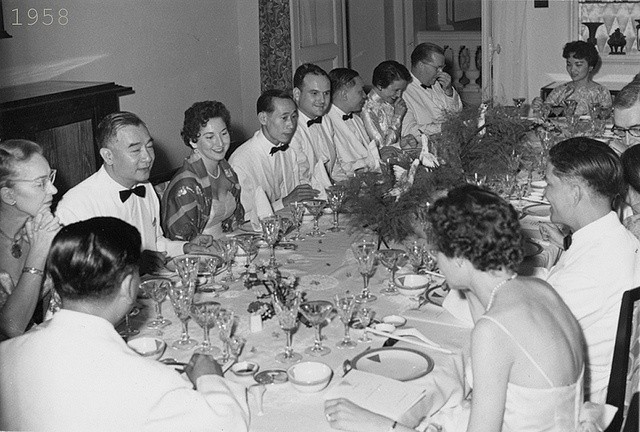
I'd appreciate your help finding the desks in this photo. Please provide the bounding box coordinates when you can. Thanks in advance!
[0,80,135,217]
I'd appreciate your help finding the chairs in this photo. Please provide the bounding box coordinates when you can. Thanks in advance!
[606,286,640,432]
[148,167,183,203]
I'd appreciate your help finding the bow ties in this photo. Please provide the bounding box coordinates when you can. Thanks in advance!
[563,230,573,250]
[343,113,353,120]
[420,84,432,89]
[307,116,322,127]
[269,144,289,156]
[119,186,146,203]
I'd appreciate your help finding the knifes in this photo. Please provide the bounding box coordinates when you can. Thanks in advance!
[519,211,527,222]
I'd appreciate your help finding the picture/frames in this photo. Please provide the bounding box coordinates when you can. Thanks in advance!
[569,0,640,62]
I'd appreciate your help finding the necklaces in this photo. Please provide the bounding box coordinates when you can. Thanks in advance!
[204,165,222,181]
[1,214,34,259]
[485,270,518,313]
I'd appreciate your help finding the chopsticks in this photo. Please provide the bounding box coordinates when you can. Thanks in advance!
[365,327,458,354]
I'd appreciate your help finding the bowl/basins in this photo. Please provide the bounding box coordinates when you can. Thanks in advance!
[287,362,333,394]
[231,245,258,264]
[394,274,430,297]
[127,336,166,360]
[300,208,315,225]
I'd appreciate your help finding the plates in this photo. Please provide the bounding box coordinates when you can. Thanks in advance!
[382,315,407,326]
[230,361,259,377]
[522,204,551,220]
[255,238,269,250]
[425,285,450,306]
[323,207,333,216]
[239,219,270,233]
[165,251,228,275]
[352,347,434,382]
[519,241,544,258]
[135,276,173,304]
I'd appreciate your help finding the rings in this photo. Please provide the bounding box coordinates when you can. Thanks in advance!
[326,413,332,422]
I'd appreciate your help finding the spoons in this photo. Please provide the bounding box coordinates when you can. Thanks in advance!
[248,383,268,418]
[396,327,440,349]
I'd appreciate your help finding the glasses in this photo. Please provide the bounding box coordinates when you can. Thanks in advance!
[611,124,640,137]
[8,168,58,191]
[421,60,446,71]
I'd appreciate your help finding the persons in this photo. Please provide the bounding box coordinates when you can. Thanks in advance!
[401,43,463,139]
[1,216,251,431]
[54,111,214,270]
[228,89,332,219]
[531,40,613,119]
[624,147,640,238]
[544,138,640,406]
[0,139,62,338]
[328,68,417,176]
[359,61,414,143]
[319,183,608,432]
[159,101,245,241]
[288,62,350,189]
[609,80,640,148]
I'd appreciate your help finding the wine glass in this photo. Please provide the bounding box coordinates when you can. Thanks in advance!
[333,294,358,349]
[261,222,280,264]
[537,110,550,124]
[279,220,293,243]
[589,102,601,124]
[237,233,261,273]
[115,313,139,338]
[300,300,332,356]
[508,158,521,183]
[167,279,198,351]
[287,203,305,243]
[227,336,247,363]
[211,309,235,367]
[599,107,611,125]
[138,279,171,329]
[352,240,377,304]
[271,290,301,364]
[551,103,563,123]
[514,98,525,120]
[406,240,424,274]
[357,307,373,344]
[378,250,401,296]
[519,156,539,192]
[188,302,221,362]
[304,201,327,237]
[502,174,517,201]
[466,173,486,186]
[200,258,222,289]
[217,238,237,282]
[174,256,201,303]
[323,186,347,234]
[485,180,501,194]
[563,100,576,122]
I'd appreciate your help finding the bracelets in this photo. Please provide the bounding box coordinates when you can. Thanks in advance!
[22,265,45,277]
[389,421,398,432]
